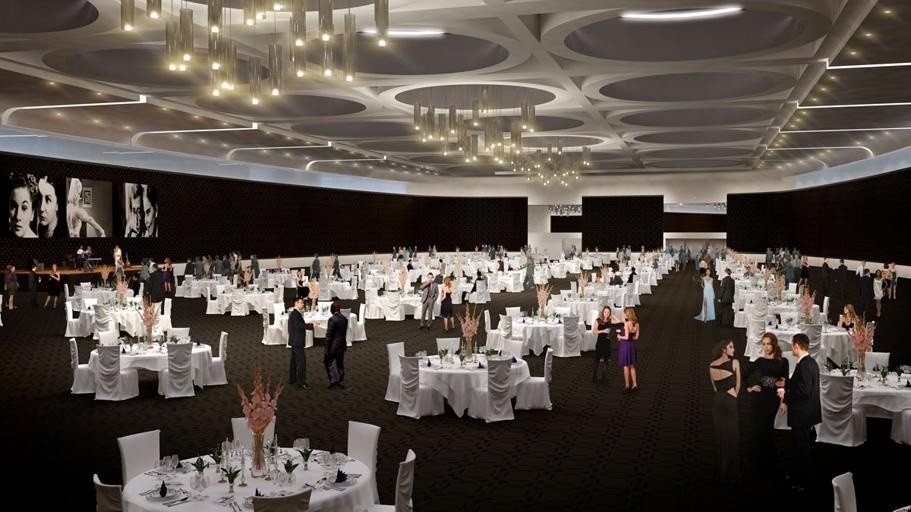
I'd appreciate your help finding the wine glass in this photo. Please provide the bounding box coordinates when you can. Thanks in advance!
[108,335,191,355]
[415,344,522,370]
[153,433,345,508]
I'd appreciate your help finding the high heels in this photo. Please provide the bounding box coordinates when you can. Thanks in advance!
[623,387,636,392]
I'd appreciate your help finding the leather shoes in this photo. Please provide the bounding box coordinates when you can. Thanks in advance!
[298,384,311,390]
[328,381,344,389]
